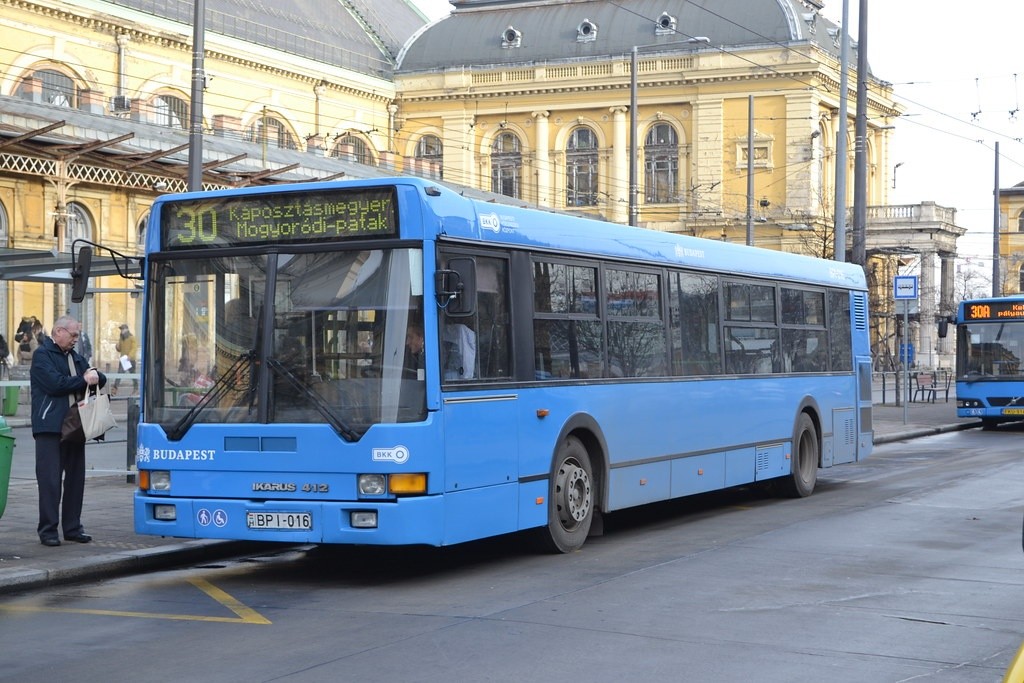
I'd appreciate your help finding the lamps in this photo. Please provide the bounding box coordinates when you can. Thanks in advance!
[153,181,167,189]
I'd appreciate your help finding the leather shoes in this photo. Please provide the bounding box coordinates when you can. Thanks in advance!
[64,533,92,543]
[41,537,60,546]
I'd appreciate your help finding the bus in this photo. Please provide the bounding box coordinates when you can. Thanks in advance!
[937,294,1024,431]
[70,176,874,560]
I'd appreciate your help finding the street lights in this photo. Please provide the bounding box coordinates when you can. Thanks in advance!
[629,37,711,230]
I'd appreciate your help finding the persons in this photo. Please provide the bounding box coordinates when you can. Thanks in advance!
[79,320,92,364]
[402,322,449,386]
[178,334,198,389]
[110,324,139,396]
[30,315,106,547]
[0,316,42,376]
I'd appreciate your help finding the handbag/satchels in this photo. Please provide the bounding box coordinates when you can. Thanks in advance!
[78,385,118,441]
[60,386,96,444]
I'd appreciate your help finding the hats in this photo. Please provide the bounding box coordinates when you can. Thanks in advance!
[118,325,128,329]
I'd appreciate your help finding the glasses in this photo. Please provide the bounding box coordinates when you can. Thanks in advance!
[60,327,78,338]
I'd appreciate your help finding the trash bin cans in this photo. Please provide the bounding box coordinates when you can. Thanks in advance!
[3,386,20,416]
[0,416,16,519]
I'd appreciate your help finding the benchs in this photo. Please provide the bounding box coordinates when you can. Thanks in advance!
[913,373,949,404]
